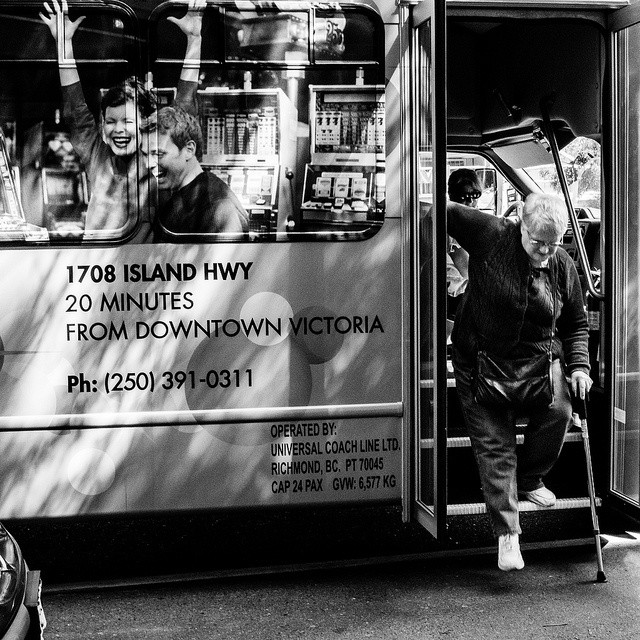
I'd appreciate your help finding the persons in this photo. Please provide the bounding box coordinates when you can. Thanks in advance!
[40,0,208,230]
[445,169,485,321]
[139,109,248,244]
[422,192,593,572]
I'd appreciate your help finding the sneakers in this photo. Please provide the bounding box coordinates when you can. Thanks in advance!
[497,534,525,570]
[518,487,557,508]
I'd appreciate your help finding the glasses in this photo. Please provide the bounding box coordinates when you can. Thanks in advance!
[454,191,481,201]
[527,229,564,247]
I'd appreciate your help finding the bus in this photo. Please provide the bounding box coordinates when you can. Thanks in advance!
[0,1,639,542]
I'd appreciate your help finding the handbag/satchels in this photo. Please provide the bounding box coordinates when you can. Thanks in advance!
[475,350,554,407]
